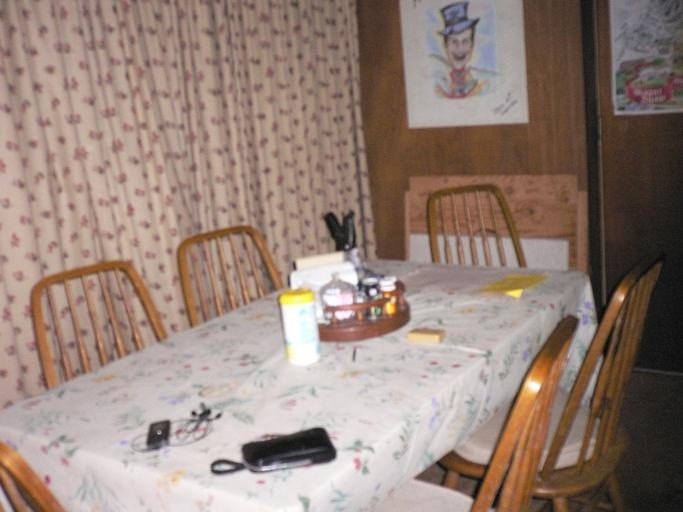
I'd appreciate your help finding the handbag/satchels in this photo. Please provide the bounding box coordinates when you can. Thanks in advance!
[212,428,337,475]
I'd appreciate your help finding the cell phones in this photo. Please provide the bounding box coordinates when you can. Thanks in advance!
[147,419,170,448]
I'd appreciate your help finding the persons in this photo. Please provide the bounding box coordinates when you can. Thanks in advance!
[430,0,484,97]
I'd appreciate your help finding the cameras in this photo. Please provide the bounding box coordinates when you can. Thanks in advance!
[241,427,335,473]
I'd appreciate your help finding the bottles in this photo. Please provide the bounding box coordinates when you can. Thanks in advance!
[380,280,399,315]
[278,287,321,367]
[361,277,383,316]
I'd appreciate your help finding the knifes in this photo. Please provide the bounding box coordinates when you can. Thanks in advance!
[323,208,357,252]
